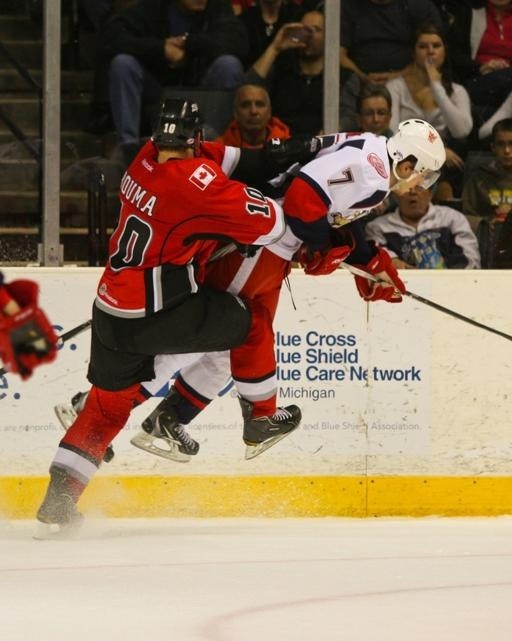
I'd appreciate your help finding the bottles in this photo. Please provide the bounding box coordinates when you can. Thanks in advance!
[176,33,188,49]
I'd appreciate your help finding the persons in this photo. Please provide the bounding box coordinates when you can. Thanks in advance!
[31,93,315,541]
[51,119,446,471]
[109,0,512,267]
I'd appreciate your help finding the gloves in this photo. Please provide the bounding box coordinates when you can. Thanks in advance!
[348,239,405,304]
[0,279,58,381]
[296,225,356,276]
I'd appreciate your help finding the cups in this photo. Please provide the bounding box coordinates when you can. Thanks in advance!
[286,27,313,44]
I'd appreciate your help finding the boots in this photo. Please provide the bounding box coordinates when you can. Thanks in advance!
[142,386,199,456]
[237,392,302,445]
[36,466,86,526]
[72,392,114,463]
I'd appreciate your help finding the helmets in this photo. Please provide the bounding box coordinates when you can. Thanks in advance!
[386,118,447,196]
[148,97,206,158]
